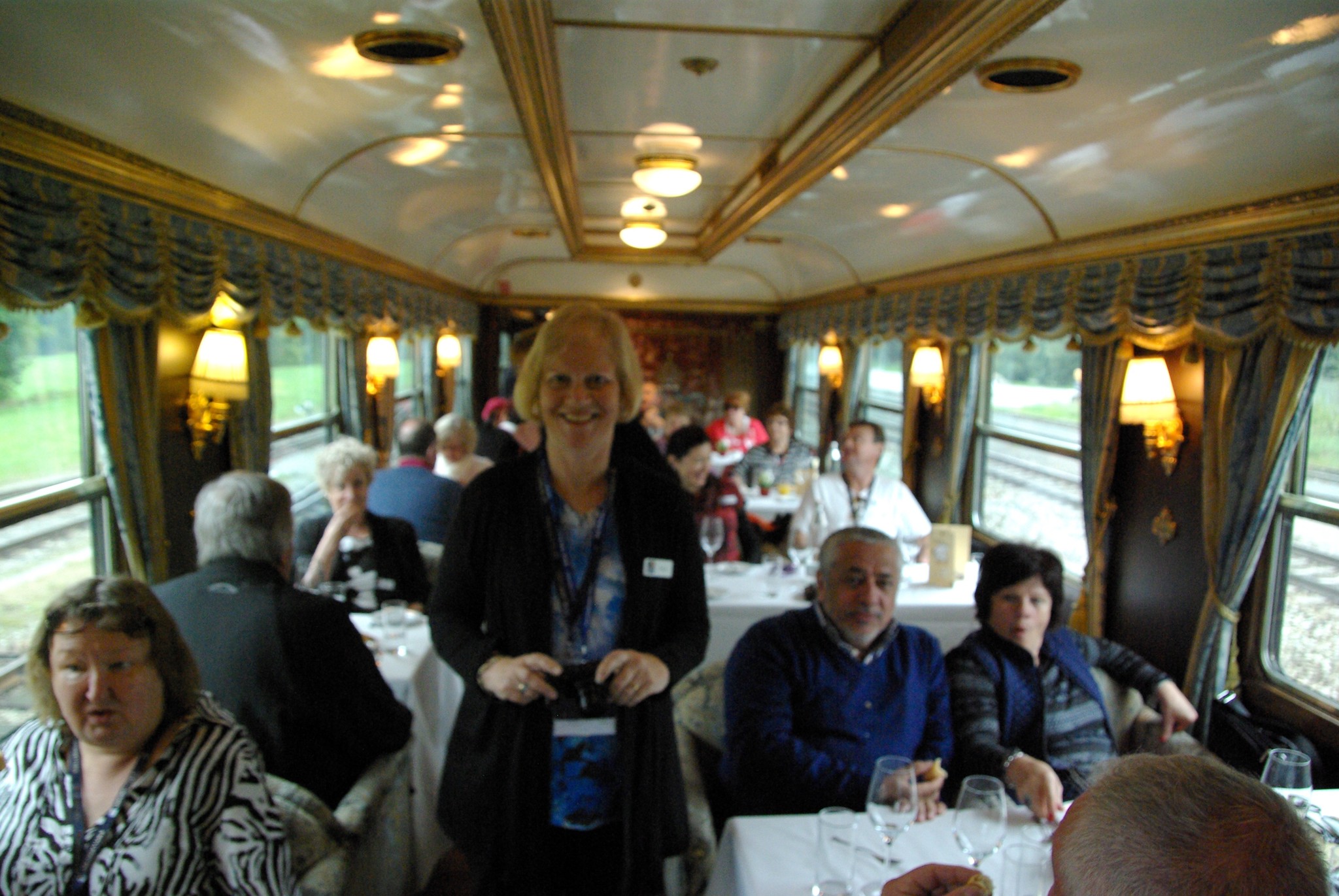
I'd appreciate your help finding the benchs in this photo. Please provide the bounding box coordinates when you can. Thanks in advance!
[656,652,1221,896]
[0,705,424,896]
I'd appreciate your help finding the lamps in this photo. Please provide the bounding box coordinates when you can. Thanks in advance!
[365,337,400,396]
[1118,356,1185,478]
[435,334,463,380]
[908,346,945,404]
[184,329,251,460]
[632,155,702,202]
[817,345,844,387]
[619,220,667,251]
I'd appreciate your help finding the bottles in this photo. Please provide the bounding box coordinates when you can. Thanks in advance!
[824,439,842,475]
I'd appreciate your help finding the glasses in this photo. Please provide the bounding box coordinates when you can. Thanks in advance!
[723,401,742,411]
[539,373,619,391]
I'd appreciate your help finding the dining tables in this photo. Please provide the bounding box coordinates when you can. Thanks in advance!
[700,557,986,667]
[737,486,803,525]
[700,788,1339,896]
[351,606,444,890]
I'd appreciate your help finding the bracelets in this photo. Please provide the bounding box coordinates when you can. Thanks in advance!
[1002,750,1028,790]
[476,653,505,695]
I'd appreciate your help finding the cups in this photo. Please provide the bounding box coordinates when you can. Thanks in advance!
[1260,746,1312,823]
[997,817,1067,895]
[809,803,856,896]
[762,550,784,596]
[381,596,413,655]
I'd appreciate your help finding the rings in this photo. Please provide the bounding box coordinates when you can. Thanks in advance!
[518,682,527,695]
[629,683,641,691]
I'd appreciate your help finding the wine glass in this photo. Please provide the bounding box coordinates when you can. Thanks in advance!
[698,513,725,570]
[948,775,1009,873]
[861,752,920,896]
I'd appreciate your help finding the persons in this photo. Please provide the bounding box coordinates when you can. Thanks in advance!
[782,417,935,564]
[707,527,958,837]
[282,438,434,616]
[727,404,822,546]
[879,756,1334,896]
[940,542,1199,822]
[0,571,306,896]
[469,373,700,467]
[429,412,498,486]
[149,467,413,808]
[363,418,465,549]
[654,425,763,565]
[702,390,771,456]
[420,301,711,896]
